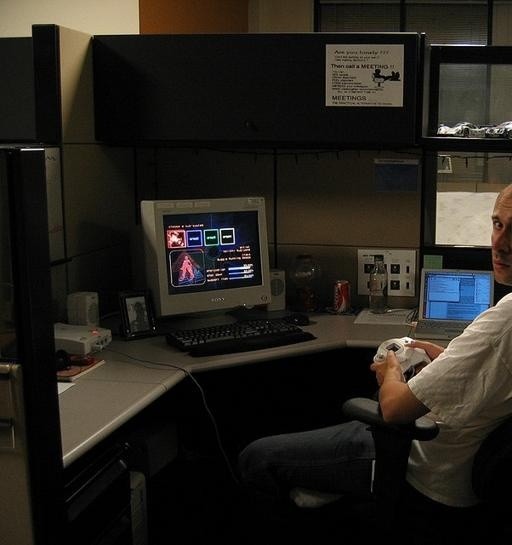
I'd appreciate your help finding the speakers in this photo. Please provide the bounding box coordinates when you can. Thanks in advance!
[65,291,100,326]
[267,267,286,311]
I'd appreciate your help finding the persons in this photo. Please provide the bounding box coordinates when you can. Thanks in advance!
[236,182,511,544]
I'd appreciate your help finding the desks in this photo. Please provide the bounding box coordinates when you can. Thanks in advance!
[51,378,168,540]
[52,310,416,388]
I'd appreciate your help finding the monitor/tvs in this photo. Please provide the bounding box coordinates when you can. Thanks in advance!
[140,195,272,318]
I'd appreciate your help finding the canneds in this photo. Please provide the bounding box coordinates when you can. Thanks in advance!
[334,279,351,314]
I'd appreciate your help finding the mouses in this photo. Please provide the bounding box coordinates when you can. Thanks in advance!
[284,312,310,326]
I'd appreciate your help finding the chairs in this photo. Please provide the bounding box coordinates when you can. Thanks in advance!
[344,395,512,545]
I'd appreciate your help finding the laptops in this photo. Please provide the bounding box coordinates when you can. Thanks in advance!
[414,267,494,340]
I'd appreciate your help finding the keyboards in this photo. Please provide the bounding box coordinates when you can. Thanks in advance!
[166,317,317,357]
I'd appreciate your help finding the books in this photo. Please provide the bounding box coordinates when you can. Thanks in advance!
[55,354,105,381]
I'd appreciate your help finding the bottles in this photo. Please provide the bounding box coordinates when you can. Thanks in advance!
[368,255,389,314]
